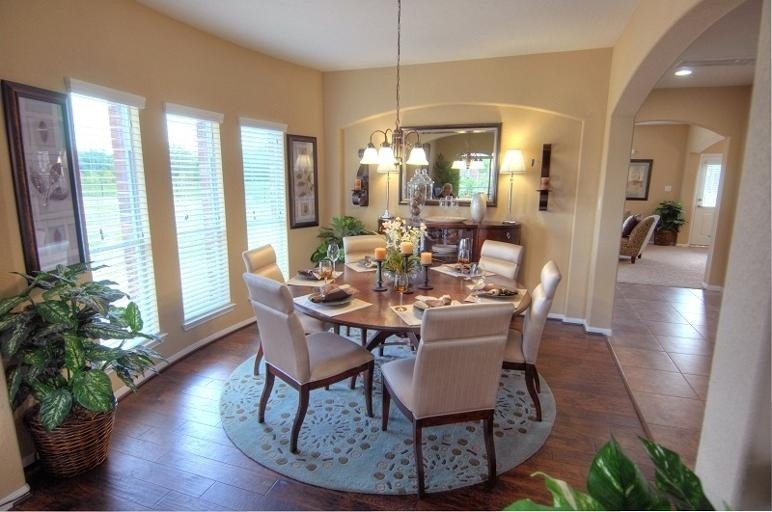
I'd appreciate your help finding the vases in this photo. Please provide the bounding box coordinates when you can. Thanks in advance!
[383,267,426,285]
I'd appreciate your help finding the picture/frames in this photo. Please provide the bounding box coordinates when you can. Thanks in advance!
[1,78,88,287]
[626,158,654,201]
[286,133,319,230]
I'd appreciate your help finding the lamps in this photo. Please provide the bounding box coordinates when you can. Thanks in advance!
[498,148,527,224]
[377,164,399,219]
[359,1,429,167]
[455,133,483,171]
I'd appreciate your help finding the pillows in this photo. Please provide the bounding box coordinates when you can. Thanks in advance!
[621,212,642,236]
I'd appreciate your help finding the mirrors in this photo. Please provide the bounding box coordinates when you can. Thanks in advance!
[397,121,503,208]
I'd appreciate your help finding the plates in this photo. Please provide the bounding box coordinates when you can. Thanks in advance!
[413,300,463,310]
[297,271,321,281]
[472,284,519,298]
[307,292,354,307]
[424,215,468,225]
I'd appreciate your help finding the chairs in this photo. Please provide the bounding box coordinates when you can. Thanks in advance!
[477,239,526,280]
[379,303,515,497]
[240,243,285,376]
[502,258,563,421]
[619,214,661,264]
[342,234,387,263]
[241,272,374,454]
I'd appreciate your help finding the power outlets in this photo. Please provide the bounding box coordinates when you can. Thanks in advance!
[664,185,672,192]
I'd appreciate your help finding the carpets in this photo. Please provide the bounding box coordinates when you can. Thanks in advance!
[218,324,558,497]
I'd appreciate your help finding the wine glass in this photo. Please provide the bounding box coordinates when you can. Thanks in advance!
[327,242,341,277]
[318,260,333,285]
[393,272,409,312]
[439,195,461,220]
[457,237,473,279]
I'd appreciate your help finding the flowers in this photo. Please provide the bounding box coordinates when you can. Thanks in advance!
[379,209,428,272]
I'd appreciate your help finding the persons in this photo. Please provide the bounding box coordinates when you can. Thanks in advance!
[438,183,454,199]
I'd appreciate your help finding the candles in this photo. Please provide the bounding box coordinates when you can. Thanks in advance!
[374,241,432,265]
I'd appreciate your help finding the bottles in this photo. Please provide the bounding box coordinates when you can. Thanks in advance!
[421,169,435,200]
[406,168,427,230]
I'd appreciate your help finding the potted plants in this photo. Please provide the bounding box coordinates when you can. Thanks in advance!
[652,199,688,246]
[1,260,171,479]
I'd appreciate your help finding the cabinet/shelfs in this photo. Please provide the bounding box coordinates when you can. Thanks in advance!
[379,212,521,263]
